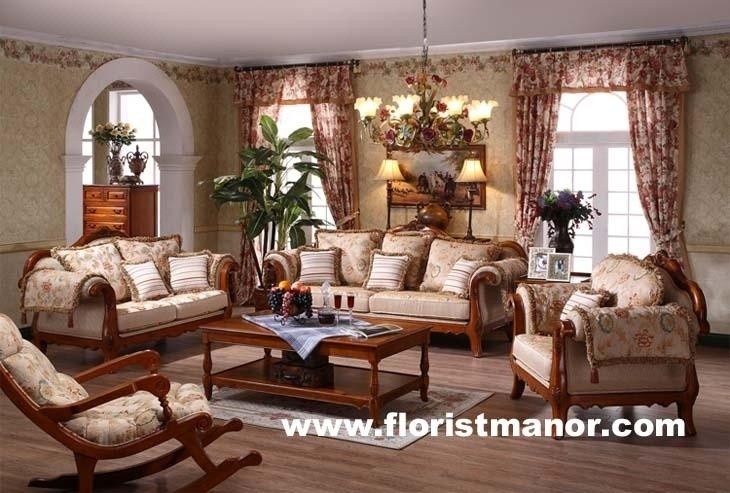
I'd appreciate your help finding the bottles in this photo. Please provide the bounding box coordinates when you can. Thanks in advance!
[318,280,335,325]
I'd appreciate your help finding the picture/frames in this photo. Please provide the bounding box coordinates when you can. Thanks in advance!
[546,252,571,283]
[527,247,556,280]
[386,144,486,210]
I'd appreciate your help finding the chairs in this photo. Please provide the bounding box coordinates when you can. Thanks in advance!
[507,251,710,439]
[0,312,262,493]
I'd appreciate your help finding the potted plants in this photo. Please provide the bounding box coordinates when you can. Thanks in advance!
[197,114,333,311]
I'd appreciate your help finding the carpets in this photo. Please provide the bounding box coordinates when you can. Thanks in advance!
[198,364,495,451]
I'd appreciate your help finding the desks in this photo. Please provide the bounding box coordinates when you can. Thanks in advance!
[451,235,491,244]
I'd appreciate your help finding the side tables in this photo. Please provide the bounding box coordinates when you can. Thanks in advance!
[511,274,591,291]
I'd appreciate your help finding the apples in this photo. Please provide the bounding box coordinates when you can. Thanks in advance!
[290,281,311,293]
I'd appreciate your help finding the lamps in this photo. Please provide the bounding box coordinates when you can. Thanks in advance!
[455,159,487,239]
[354,0,500,156]
[374,159,405,231]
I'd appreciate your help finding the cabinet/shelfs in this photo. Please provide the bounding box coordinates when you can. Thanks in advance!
[82,184,159,238]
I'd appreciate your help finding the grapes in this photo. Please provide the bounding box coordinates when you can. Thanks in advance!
[267,289,313,319]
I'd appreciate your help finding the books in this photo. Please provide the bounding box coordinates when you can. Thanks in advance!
[339,323,403,338]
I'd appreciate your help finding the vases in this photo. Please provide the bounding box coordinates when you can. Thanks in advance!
[415,200,451,231]
[553,220,573,254]
[105,150,126,184]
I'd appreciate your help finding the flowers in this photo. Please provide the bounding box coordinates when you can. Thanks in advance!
[87,122,137,152]
[529,189,601,237]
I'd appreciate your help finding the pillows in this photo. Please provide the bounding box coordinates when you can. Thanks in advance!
[591,254,665,307]
[47,234,213,304]
[561,286,611,320]
[295,227,501,299]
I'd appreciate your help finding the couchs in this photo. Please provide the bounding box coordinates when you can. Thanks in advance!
[262,225,527,359]
[18,226,239,373]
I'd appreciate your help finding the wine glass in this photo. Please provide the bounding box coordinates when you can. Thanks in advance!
[334,294,354,329]
